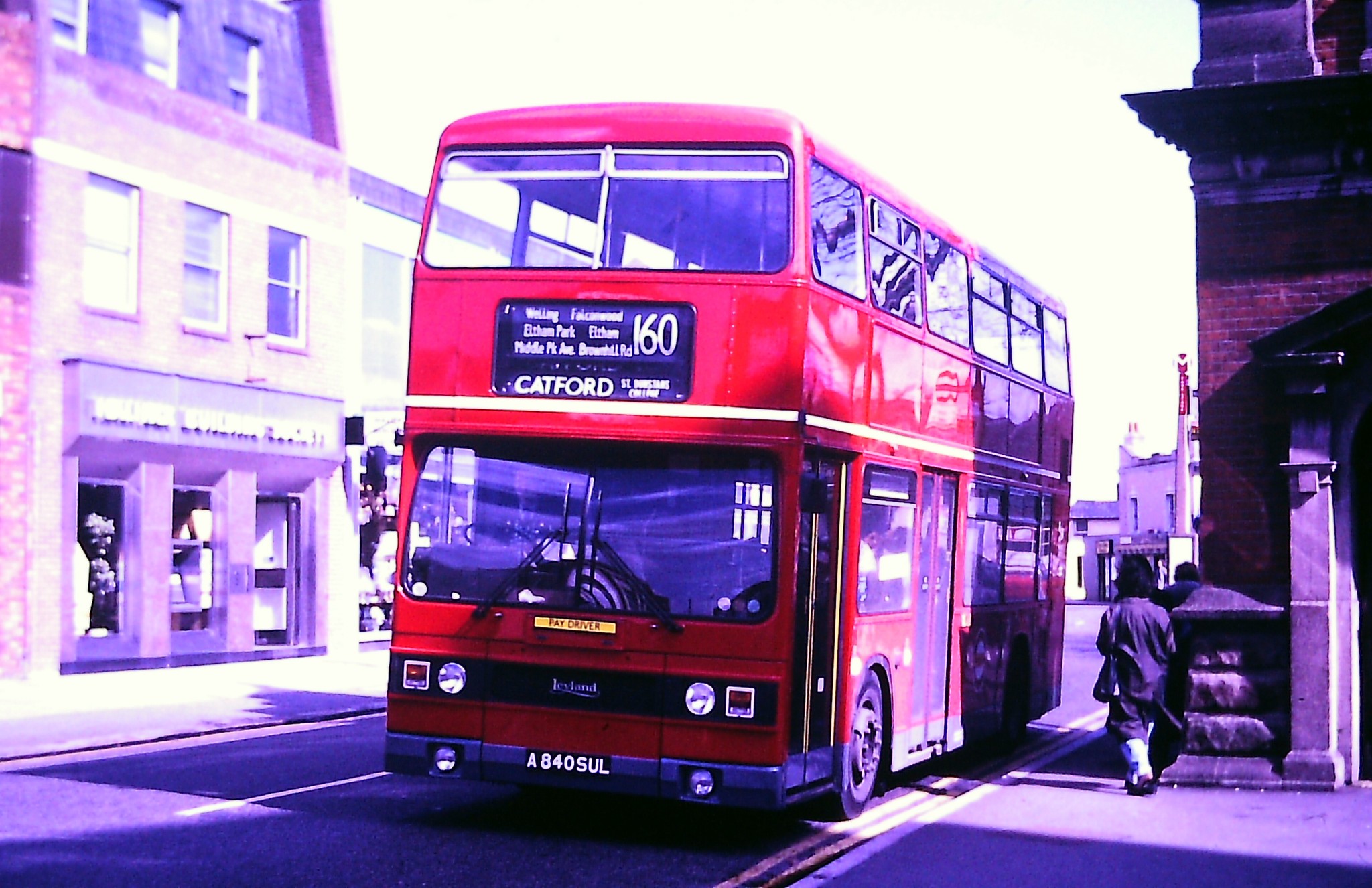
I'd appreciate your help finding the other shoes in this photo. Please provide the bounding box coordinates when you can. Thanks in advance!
[1127,777,1159,795]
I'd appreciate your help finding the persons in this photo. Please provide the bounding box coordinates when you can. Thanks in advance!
[1163,561,1202,596]
[1094,559,1177,796]
[858,520,947,615]
[473,458,556,557]
[1114,553,1190,764]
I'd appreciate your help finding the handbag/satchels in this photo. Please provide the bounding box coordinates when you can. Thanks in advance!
[1093,654,1120,702]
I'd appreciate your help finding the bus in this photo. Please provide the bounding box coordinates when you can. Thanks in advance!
[386,98,1073,811]
